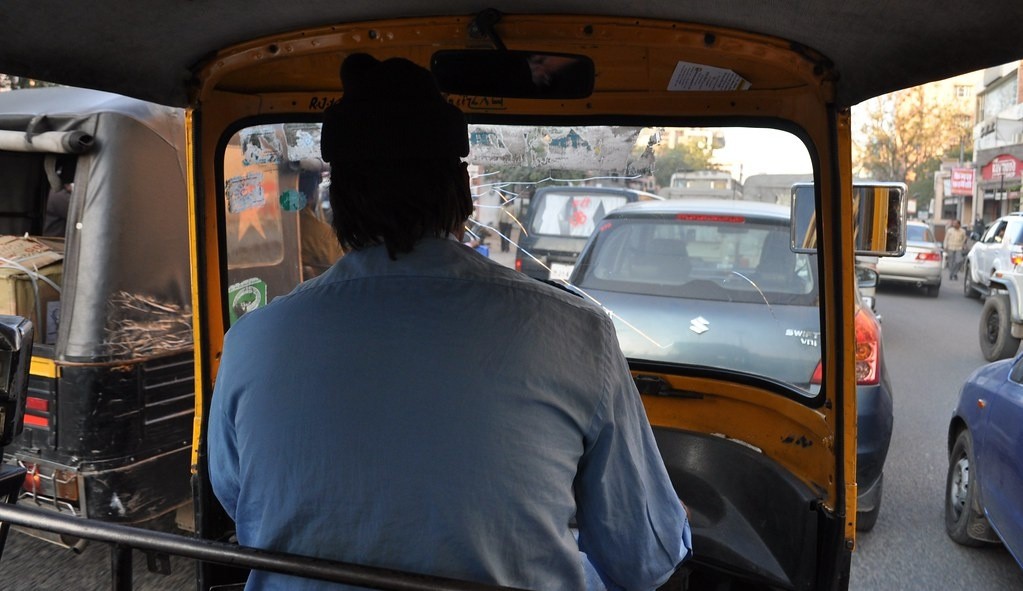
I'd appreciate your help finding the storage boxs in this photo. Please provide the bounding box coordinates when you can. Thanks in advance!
[0,233,67,347]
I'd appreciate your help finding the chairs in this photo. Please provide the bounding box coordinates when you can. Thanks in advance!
[753,231,806,293]
[639,239,692,282]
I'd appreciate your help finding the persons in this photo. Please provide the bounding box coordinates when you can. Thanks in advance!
[527,54,578,86]
[497,196,517,251]
[943,220,966,280]
[300,172,344,270]
[463,206,490,247]
[999,224,1006,236]
[974,214,984,237]
[207,54,692,590]
[43,183,71,239]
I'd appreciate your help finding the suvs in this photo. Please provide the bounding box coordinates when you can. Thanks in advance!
[979,259,1023,362]
[963,212,1023,303]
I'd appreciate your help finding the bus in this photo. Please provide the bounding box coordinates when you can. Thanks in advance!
[669,172,744,190]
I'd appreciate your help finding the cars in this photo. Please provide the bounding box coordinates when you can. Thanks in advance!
[875,221,942,296]
[564,197,895,535]
[944,351,1022,571]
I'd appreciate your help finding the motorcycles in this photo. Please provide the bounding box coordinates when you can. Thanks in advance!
[0,85,344,577]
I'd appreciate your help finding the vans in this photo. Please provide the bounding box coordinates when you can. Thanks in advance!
[515,186,640,283]
[656,186,743,200]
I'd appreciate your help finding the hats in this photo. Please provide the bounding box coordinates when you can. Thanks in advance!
[322,52,469,162]
